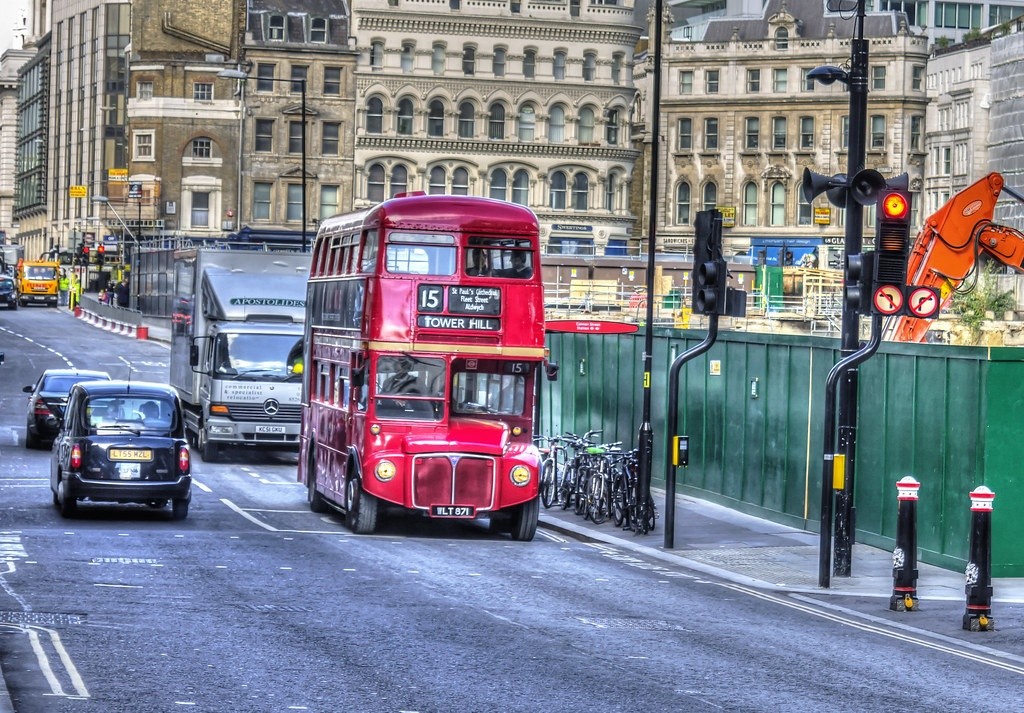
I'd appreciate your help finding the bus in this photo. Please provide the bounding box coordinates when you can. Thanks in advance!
[299,189,561,542]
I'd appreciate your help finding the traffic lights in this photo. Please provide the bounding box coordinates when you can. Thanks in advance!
[96,246,105,266]
[671,436,690,469]
[841,251,873,317]
[73,242,84,265]
[694,261,729,317]
[832,453,847,492]
[871,187,942,320]
[82,247,89,267]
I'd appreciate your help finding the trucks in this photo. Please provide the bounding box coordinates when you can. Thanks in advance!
[168,244,315,464]
[15,257,66,308]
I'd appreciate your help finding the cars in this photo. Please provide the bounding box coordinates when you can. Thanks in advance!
[21,367,126,449]
[0,277,17,310]
[50,379,193,522]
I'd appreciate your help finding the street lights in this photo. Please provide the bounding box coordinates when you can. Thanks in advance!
[805,0,873,579]
[92,195,143,314]
[213,65,309,255]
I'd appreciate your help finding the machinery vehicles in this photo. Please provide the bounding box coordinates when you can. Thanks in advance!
[880,171,1024,348]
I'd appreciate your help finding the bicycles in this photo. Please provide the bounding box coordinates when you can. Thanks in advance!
[531,428,658,535]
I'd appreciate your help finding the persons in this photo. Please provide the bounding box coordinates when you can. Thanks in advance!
[144,401,156,419]
[500,250,531,279]
[73,274,80,304]
[59,274,69,306]
[119,279,128,306]
[378,359,427,408]
[99,281,114,305]
[468,248,488,276]
